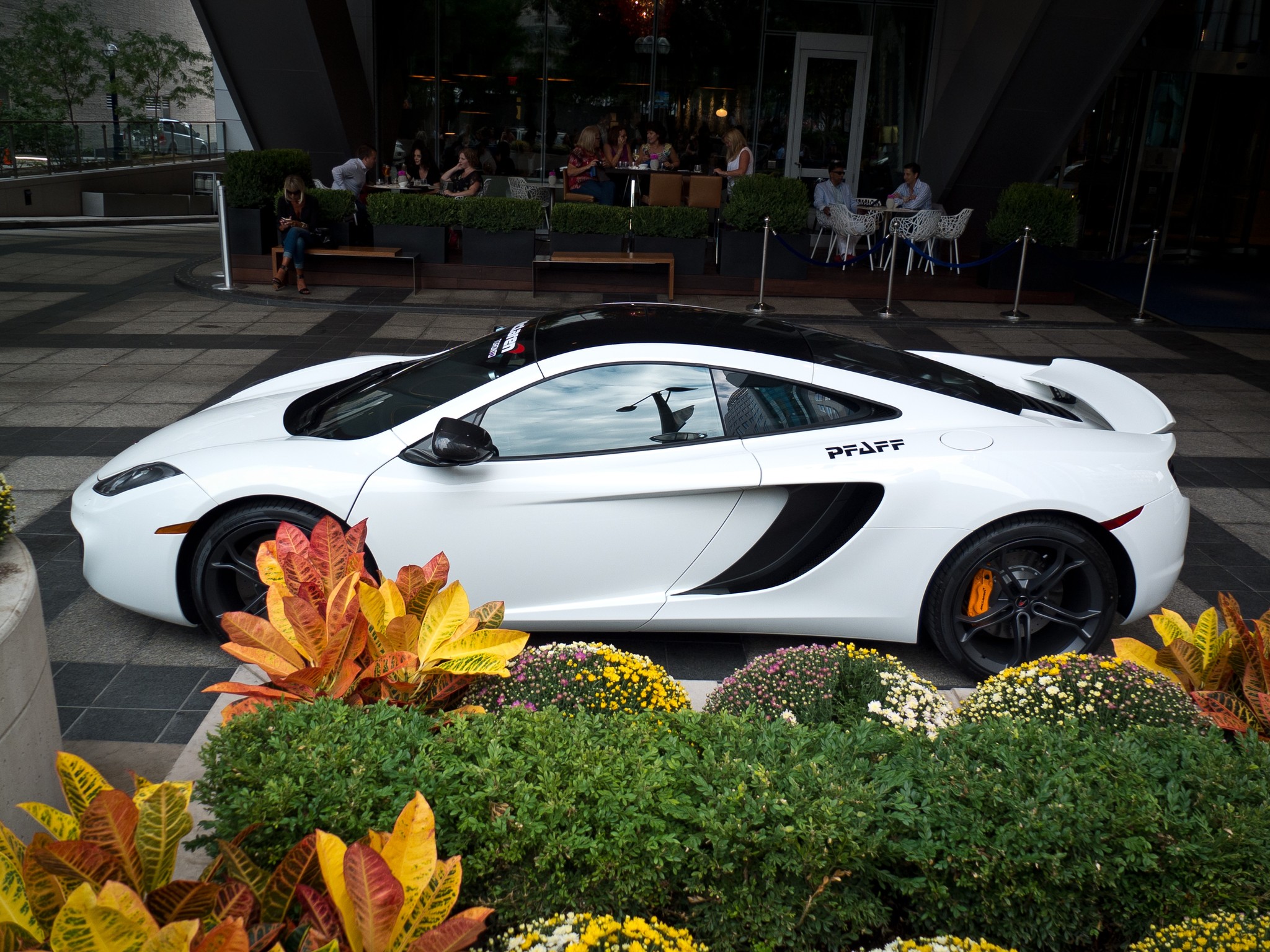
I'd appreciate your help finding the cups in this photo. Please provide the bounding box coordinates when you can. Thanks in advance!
[415,179,420,185]
[694,165,701,172]
[623,161,630,168]
[376,179,383,186]
[421,179,427,185]
[659,162,666,170]
[398,181,408,187]
[633,149,639,161]
[617,161,623,168]
[630,162,637,168]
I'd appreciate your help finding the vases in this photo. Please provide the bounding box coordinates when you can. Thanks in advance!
[548,176,556,185]
[886,198,895,209]
[398,175,407,183]
[650,159,659,170]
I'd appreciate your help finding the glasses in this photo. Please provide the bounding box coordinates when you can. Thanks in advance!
[832,171,846,175]
[286,189,300,196]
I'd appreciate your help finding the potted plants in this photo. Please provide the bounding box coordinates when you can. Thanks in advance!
[225,148,358,257]
[366,192,545,270]
[977,182,1082,291]
[718,174,810,280]
[550,202,710,276]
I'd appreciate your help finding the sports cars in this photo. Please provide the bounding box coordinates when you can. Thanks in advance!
[68,300,1190,686]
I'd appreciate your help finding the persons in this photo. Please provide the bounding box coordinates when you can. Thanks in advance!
[676,120,712,176]
[595,108,647,207]
[634,122,679,206]
[736,123,813,168]
[4,144,12,167]
[271,174,321,295]
[397,96,425,155]
[873,162,931,271]
[406,147,481,197]
[331,144,378,246]
[442,124,516,176]
[814,163,867,262]
[713,127,754,222]
[567,125,616,206]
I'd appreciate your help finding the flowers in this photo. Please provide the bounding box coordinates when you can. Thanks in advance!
[887,194,895,198]
[549,172,556,176]
[398,171,406,176]
[650,153,659,160]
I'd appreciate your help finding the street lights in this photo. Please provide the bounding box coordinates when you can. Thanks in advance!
[103,44,129,160]
[520,1,569,182]
[636,6,673,128]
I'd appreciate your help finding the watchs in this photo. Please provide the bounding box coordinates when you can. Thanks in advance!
[725,171,728,176]
[301,222,307,229]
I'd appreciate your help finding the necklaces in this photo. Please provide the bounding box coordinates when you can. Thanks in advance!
[462,168,472,177]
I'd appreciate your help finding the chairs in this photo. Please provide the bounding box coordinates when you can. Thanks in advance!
[508,168,722,266]
[810,198,974,275]
[311,177,493,227]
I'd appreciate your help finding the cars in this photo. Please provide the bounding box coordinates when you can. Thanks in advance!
[508,127,541,149]
[124,118,209,154]
[555,133,573,147]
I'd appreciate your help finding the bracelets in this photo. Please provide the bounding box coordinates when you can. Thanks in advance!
[722,171,724,176]
[607,163,609,166]
[671,163,674,170]
[616,152,620,156]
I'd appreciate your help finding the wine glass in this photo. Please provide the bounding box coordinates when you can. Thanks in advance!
[382,164,390,186]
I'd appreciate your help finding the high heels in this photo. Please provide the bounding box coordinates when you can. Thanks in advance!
[272,265,287,285]
[296,274,310,295]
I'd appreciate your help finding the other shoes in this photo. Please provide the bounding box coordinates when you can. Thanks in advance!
[845,254,856,261]
[832,255,843,264]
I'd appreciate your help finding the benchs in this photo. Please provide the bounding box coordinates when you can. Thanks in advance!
[532,252,674,301]
[272,245,423,295]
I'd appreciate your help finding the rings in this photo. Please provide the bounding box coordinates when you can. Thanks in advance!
[291,225,292,227]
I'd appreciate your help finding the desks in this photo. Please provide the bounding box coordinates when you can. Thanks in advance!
[521,183,563,231]
[854,206,921,269]
[365,184,440,193]
[606,167,703,253]
